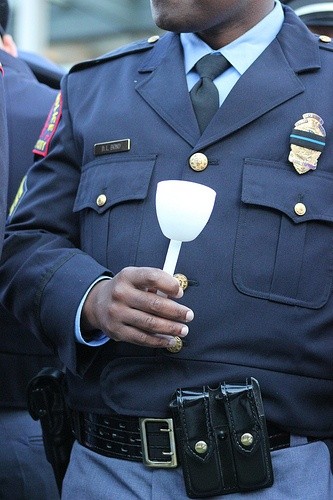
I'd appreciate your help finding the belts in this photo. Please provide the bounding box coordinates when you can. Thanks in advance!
[68,403,318,469]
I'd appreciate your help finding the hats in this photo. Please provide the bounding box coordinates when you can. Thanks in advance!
[287,0,333,29]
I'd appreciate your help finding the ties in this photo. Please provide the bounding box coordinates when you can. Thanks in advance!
[188,54,229,135]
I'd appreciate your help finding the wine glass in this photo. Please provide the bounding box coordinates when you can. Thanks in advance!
[145,179,218,341]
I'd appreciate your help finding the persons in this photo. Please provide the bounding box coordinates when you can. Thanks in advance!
[0,0,333,500]
[0,0,65,90]
[283,0,333,37]
[0,65,68,500]
